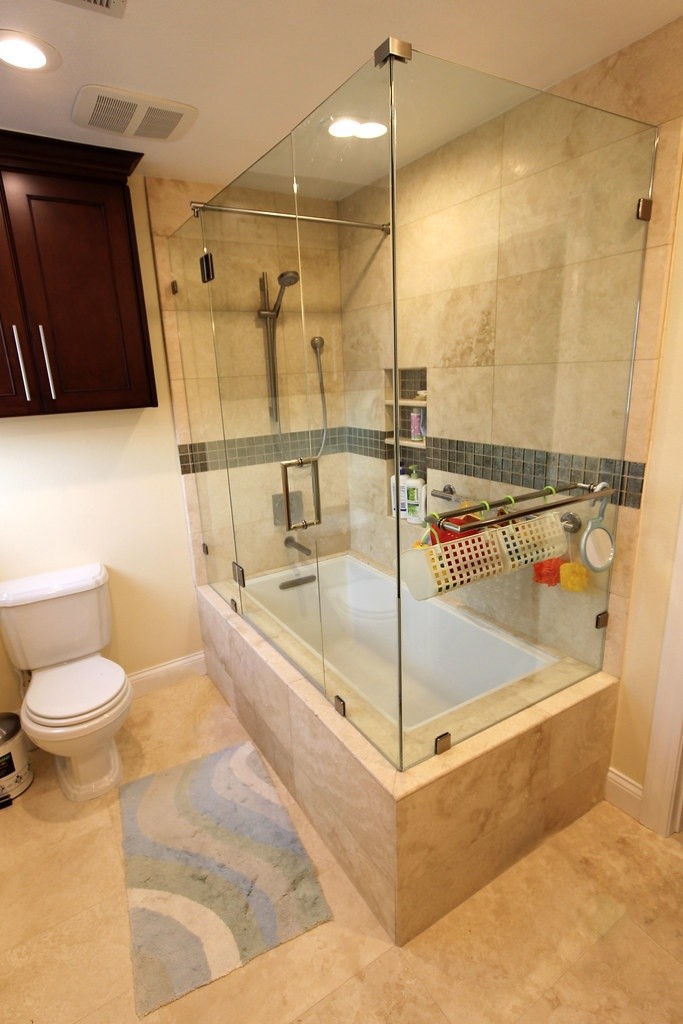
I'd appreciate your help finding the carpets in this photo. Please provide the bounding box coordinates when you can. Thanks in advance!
[117,739,334,1020]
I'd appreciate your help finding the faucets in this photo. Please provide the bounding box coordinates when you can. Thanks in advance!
[284,535,313,557]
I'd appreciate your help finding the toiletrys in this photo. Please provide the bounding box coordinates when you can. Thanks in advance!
[420,484,430,529]
[390,460,413,520]
[418,390,428,448]
[410,389,423,444]
[405,464,427,525]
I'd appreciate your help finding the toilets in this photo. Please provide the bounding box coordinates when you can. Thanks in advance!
[1,563,136,805]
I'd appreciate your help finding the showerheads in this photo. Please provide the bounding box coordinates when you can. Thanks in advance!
[271,270,300,319]
[310,335,326,362]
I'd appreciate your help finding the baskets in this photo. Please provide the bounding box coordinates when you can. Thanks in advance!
[400,509,568,601]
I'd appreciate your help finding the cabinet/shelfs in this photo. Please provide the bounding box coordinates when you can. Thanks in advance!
[0,157,160,419]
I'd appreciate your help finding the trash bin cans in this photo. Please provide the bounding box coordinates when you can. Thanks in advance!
[0,711,34,809]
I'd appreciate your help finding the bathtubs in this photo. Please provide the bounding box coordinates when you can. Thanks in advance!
[199,550,621,950]
